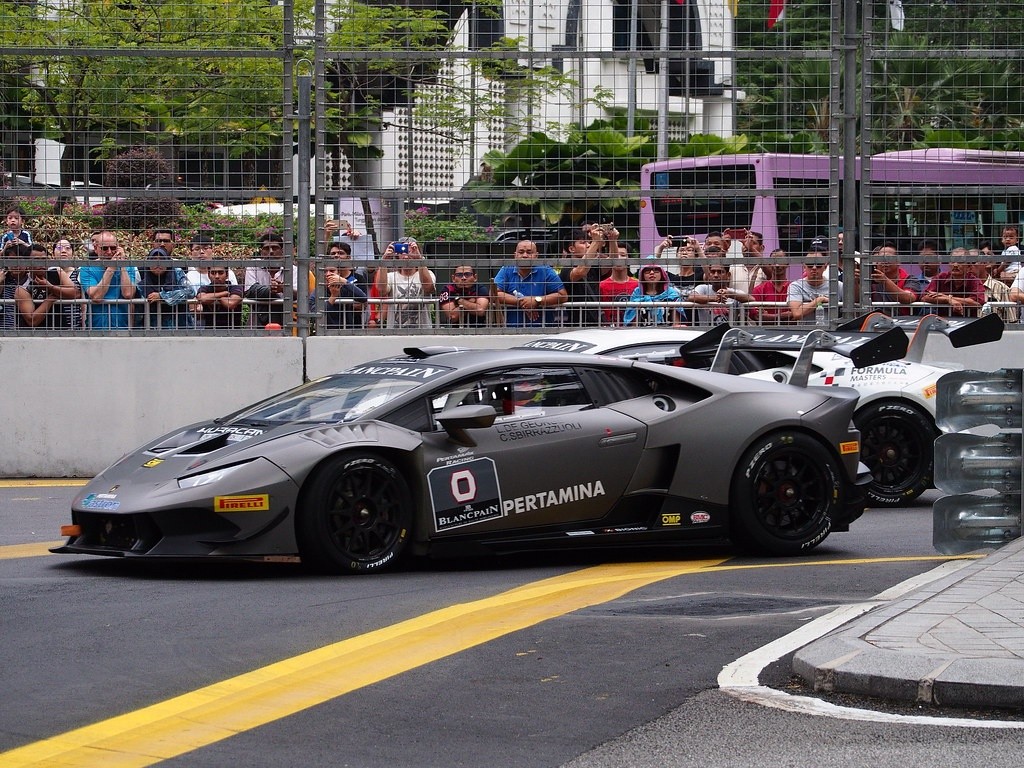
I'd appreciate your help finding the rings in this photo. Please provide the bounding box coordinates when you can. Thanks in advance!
[523,305,525,308]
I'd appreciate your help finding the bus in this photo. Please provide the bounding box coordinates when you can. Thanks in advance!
[639,144,1024,313]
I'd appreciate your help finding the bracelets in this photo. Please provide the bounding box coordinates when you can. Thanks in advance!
[50,285,54,291]
[948,295,953,298]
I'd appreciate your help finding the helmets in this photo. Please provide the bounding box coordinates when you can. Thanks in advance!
[502,372,554,415]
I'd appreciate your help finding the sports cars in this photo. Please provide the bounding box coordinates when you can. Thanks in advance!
[500,307,1007,508]
[47,322,910,580]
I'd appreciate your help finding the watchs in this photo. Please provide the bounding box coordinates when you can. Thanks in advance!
[535,295,542,305]
[459,304,464,310]
[455,296,460,305]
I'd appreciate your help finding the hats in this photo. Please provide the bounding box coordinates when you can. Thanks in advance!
[811,235,829,248]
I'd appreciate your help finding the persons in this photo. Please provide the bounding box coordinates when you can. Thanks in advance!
[491,368,555,423]
[0,201,1024,333]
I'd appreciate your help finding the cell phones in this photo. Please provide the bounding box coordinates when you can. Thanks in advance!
[857,264,877,274]
[327,220,348,230]
[393,243,408,254]
[599,224,613,232]
[727,229,747,239]
[669,236,687,247]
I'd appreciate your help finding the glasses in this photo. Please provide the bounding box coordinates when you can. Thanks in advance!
[192,247,212,251]
[155,239,172,243]
[676,249,694,254]
[97,245,118,251]
[805,263,824,268]
[642,267,662,273]
[455,272,475,279]
[56,245,71,251]
